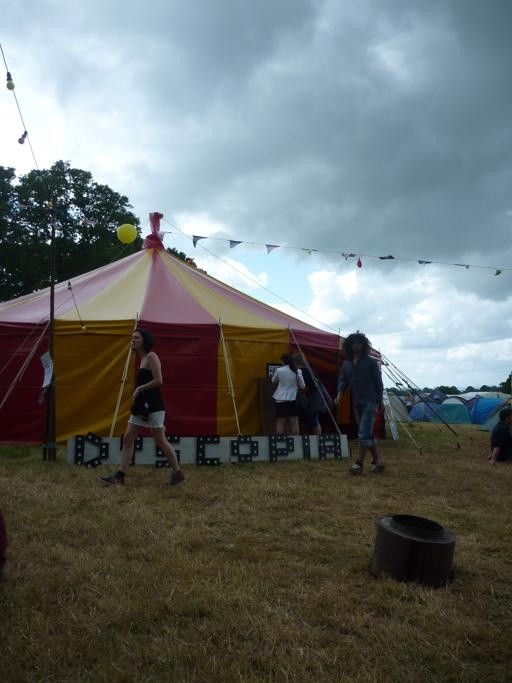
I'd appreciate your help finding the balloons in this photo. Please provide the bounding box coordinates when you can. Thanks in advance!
[115,221,138,244]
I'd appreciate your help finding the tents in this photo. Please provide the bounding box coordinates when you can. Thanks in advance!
[1,206,401,443]
[395,388,511,432]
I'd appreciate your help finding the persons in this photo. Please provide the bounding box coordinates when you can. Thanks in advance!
[268,352,307,436]
[100,327,187,488]
[289,352,323,435]
[330,331,387,474]
[482,407,512,464]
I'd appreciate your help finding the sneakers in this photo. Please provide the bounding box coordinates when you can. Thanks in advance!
[100,474,125,486]
[164,470,185,489]
[350,459,364,474]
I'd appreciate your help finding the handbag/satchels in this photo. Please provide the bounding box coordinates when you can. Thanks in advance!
[130,396,150,418]
